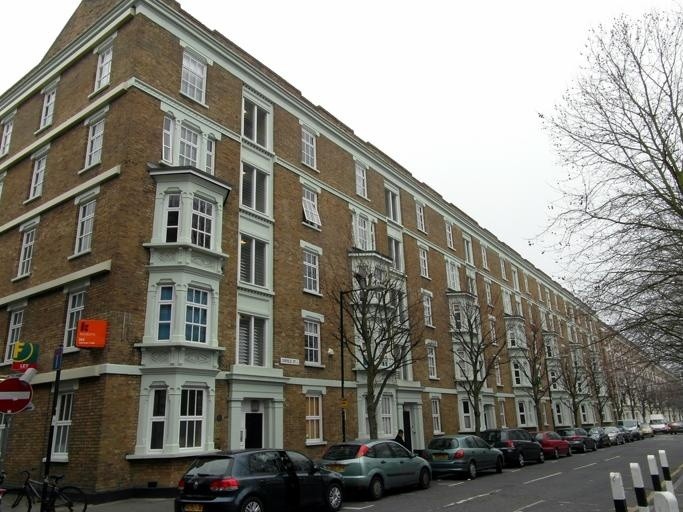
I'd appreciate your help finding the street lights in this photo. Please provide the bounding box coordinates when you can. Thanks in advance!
[543,354,567,432]
[339,284,386,441]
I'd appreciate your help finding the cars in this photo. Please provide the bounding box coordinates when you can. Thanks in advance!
[583,427,611,449]
[668,422,682,435]
[617,426,631,443]
[422,434,505,481]
[527,431,571,460]
[639,423,654,439]
[316,439,433,501]
[602,427,625,445]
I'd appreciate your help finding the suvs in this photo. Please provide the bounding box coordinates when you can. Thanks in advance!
[479,428,545,469]
[173,448,346,511]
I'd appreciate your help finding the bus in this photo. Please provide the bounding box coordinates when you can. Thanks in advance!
[555,428,597,453]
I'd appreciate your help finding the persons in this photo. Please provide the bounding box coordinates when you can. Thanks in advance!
[394,430,404,446]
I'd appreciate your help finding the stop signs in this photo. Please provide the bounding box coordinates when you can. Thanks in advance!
[0,377,33,415]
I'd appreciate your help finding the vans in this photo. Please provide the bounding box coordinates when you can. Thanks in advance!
[649,413,668,435]
[622,419,641,441]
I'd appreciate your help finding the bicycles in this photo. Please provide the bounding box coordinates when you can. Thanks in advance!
[0,470,88,512]
